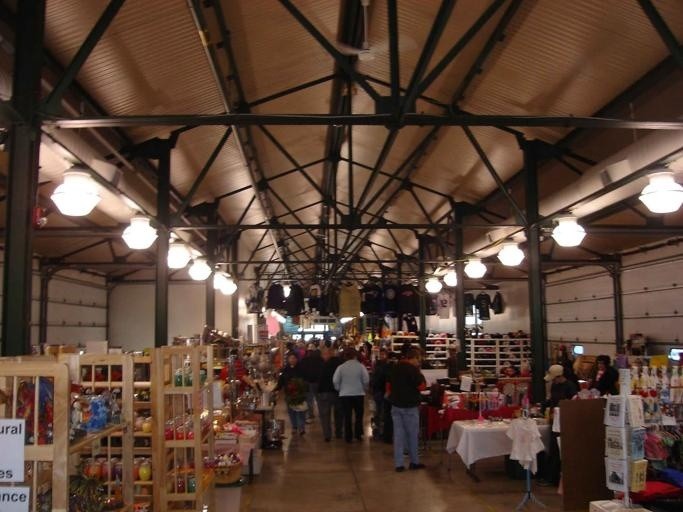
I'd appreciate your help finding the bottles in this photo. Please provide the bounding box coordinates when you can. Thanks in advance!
[534,401,541,416]
[543,407,550,425]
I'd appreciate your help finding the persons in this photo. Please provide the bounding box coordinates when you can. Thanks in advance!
[544,365,579,493]
[588,355,619,394]
[277,338,427,472]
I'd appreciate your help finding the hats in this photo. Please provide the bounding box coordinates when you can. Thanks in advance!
[543,364,563,382]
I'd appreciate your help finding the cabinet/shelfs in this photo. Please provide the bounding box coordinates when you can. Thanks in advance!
[357,329,534,389]
[199,327,275,511]
[2,351,135,512]
[86,341,214,509]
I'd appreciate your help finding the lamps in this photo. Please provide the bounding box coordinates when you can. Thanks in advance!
[424,277,441,293]
[548,214,588,248]
[636,166,683,214]
[47,164,104,217]
[496,240,525,267]
[462,256,487,279]
[119,210,159,250]
[443,270,456,287]
[169,239,237,296]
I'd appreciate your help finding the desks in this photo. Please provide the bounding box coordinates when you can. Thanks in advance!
[423,403,520,466]
[452,418,559,510]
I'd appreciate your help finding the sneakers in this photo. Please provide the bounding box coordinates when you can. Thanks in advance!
[408,461,426,469]
